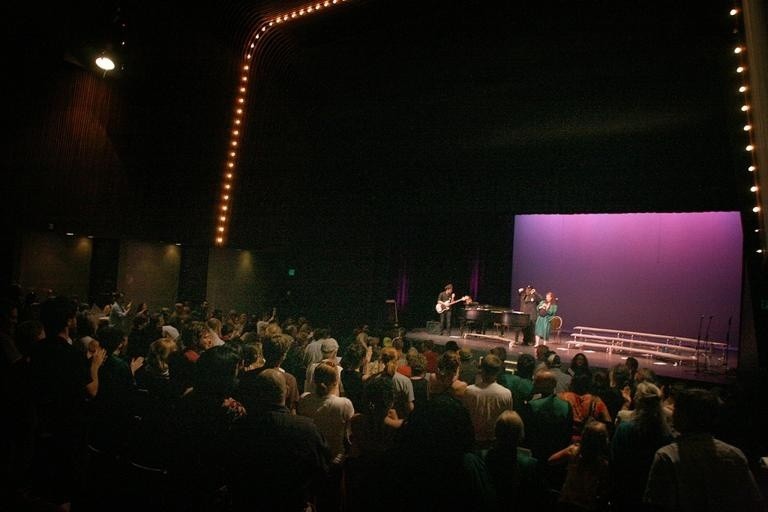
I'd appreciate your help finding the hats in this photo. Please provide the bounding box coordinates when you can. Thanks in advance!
[546,354,561,367]
[445,284,451,289]
[458,347,473,359]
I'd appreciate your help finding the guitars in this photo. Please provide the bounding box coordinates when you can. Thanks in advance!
[436,295,469,313]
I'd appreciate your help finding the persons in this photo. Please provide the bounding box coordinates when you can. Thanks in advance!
[1,284,767,511]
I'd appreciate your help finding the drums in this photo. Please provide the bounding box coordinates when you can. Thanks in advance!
[503,312,530,328]
[491,309,512,325]
[465,308,480,320]
[478,305,493,319]
